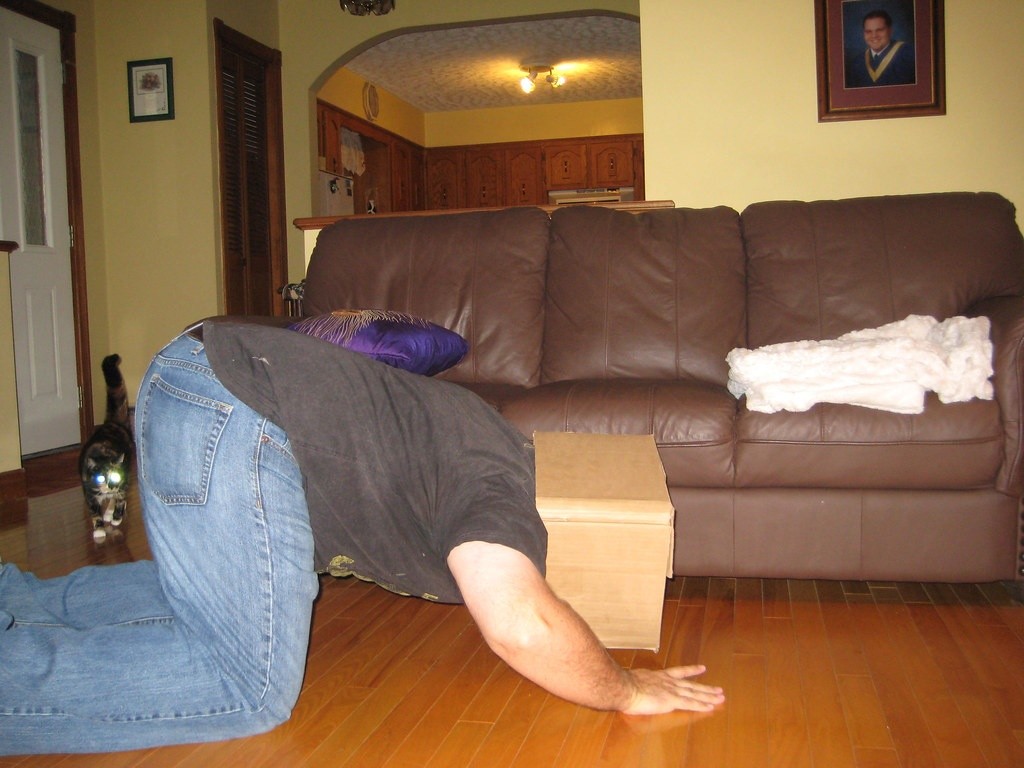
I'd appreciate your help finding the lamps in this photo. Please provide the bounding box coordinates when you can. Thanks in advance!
[519,55,564,95]
[339,0,397,17]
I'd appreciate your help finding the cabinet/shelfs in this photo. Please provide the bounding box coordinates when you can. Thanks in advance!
[312,98,645,217]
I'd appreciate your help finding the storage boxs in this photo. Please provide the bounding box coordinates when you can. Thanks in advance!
[531,429,675,653]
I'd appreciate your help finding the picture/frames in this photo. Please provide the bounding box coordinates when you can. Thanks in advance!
[814,0,947,125]
[126,56,175,125]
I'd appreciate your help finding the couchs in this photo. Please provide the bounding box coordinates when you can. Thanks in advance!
[303,189,1024,583]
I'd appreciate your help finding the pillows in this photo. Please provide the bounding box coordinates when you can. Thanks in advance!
[280,306,470,378]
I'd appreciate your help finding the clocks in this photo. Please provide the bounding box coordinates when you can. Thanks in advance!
[362,81,380,121]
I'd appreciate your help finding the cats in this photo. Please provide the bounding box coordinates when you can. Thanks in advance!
[78,352,131,538]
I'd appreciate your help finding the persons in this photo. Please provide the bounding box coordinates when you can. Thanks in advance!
[0,320,725,756]
